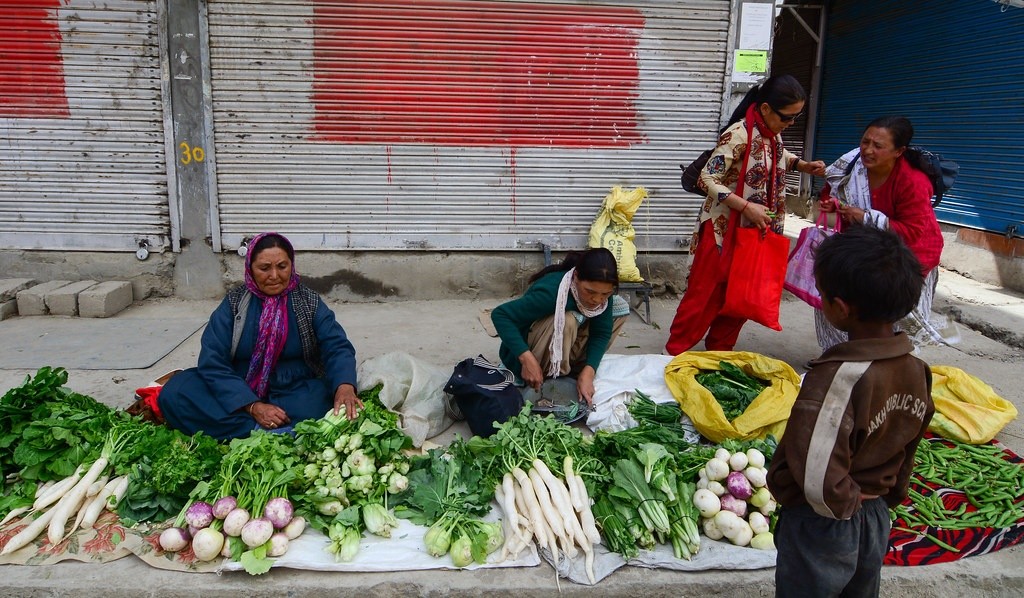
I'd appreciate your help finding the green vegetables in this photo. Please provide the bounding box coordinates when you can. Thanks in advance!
[290,383,412,562]
[116,429,231,523]
[581,424,702,561]
[0,365,147,522]
[695,360,769,423]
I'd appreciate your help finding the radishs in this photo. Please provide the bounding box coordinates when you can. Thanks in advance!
[159,498,305,560]
[0,457,128,555]
[494,456,601,590]
[693,447,775,550]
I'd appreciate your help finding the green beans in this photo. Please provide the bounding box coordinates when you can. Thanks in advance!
[887,438,1024,552]
[627,387,685,438]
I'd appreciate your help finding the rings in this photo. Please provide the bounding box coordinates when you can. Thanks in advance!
[269,422,274,426]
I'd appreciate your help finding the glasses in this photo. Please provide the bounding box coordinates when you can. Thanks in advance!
[771,106,803,123]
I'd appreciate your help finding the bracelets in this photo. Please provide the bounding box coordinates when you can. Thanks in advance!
[793,157,801,171]
[741,201,749,213]
[250,403,255,416]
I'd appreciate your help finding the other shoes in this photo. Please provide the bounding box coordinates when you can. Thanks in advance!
[499,362,524,387]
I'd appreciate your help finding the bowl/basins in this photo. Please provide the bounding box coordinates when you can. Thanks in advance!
[521,376,588,425]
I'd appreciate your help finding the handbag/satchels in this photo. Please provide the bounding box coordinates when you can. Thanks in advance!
[441,354,525,438]
[783,198,843,308]
[679,149,714,197]
[903,146,960,208]
[719,225,790,331]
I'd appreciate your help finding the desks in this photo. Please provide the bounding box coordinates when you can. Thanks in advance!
[618,282,650,326]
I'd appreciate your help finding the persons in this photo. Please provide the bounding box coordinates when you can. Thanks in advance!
[156,231,364,445]
[491,247,630,407]
[803,115,944,368]
[765,217,936,598]
[661,72,827,356]
